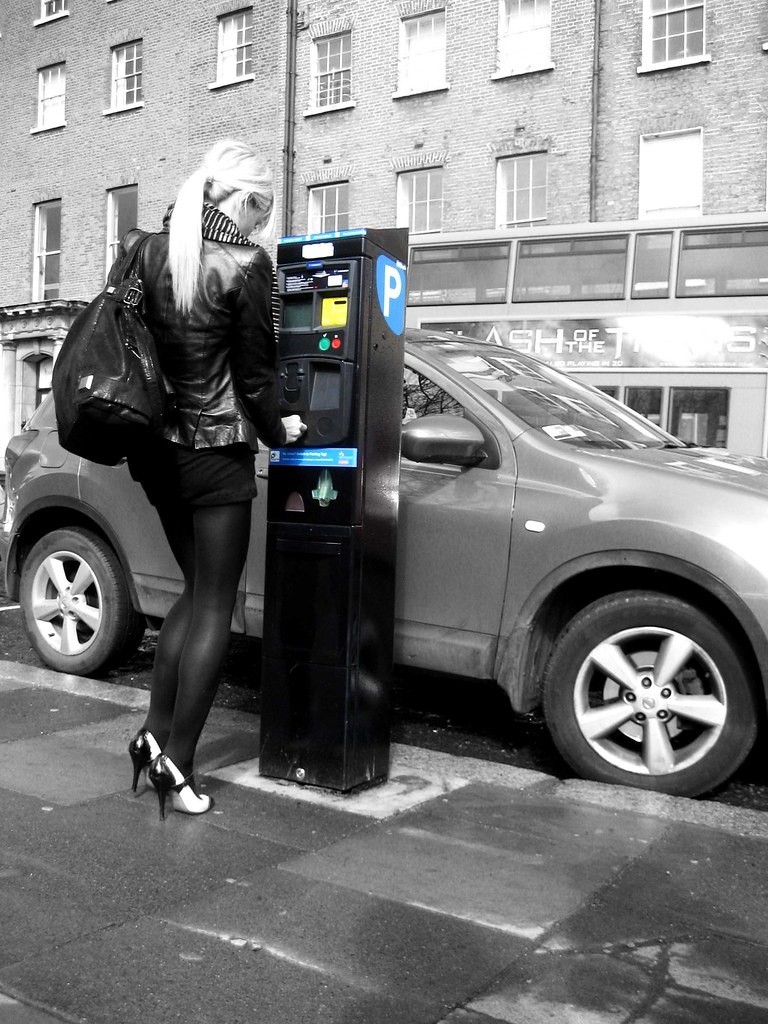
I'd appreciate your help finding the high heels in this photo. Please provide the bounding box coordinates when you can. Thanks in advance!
[149,752,215,821]
[129,727,162,794]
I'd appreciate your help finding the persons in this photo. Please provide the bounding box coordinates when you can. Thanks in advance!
[117,137,307,822]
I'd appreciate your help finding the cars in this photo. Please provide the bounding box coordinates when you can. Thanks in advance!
[1,327,767,799]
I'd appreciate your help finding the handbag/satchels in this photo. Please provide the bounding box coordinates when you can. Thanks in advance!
[50,227,165,468]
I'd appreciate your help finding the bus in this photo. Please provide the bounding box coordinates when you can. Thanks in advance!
[408,205,768,457]
